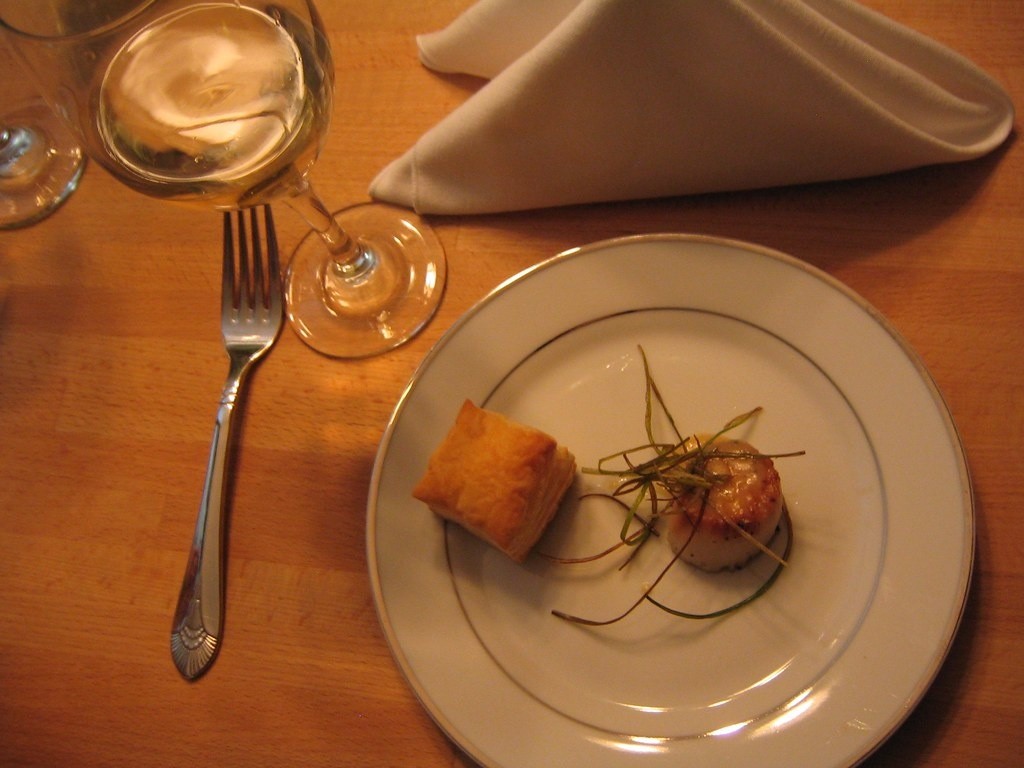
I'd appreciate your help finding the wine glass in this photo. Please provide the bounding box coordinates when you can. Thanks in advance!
[0,98,88,230]
[0,0,447,362]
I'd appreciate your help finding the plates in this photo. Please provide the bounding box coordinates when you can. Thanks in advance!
[365,232,977,768]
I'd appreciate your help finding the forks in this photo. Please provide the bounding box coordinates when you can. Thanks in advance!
[169,204,287,680]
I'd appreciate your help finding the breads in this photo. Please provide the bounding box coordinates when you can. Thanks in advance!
[412,397,577,562]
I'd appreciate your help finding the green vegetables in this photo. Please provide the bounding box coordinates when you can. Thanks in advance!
[533,345,796,625]
